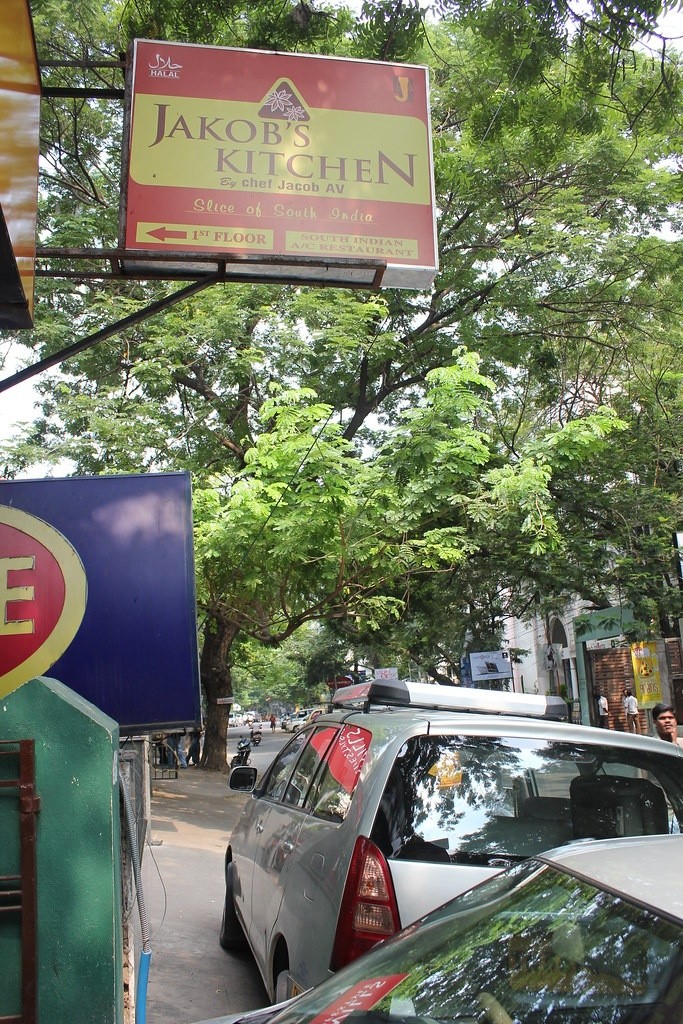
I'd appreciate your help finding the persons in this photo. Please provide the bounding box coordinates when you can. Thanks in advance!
[620,687,635,732]
[597,692,612,729]
[152,729,203,769]
[249,718,262,741]
[269,714,276,733]
[624,689,642,735]
[645,702,683,835]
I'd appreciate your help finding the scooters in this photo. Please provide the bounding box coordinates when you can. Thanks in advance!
[250,726,261,746]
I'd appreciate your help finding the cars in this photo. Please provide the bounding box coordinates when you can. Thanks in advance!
[228,714,244,727]
[180,833,679,1024]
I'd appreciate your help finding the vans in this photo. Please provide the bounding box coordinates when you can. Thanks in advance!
[288,709,316,733]
[307,708,344,726]
[220,680,681,1003]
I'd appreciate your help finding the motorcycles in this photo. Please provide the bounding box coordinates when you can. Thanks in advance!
[231,735,254,769]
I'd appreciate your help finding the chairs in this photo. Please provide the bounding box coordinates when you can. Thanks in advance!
[497,795,577,858]
